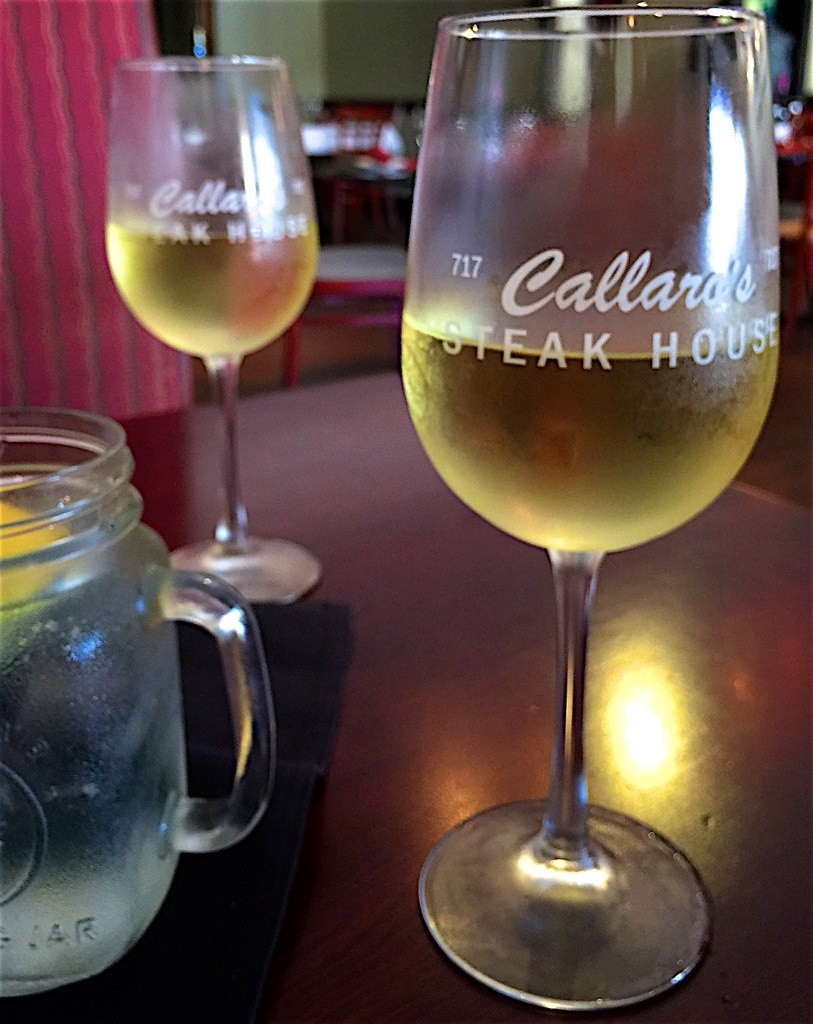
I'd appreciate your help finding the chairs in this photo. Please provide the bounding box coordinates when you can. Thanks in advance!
[282,245,411,389]
[322,109,400,245]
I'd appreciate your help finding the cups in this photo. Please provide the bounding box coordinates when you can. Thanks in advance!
[0,396,279,1001]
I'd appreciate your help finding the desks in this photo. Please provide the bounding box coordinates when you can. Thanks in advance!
[0,372,813,1024]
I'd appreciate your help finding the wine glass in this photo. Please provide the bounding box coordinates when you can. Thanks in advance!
[401,6,783,1016]
[103,57,322,611]
